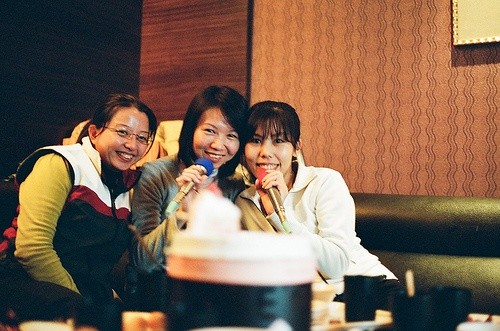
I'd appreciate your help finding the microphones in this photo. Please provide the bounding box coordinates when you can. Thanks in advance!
[165,158,214,218]
[258,168,292,234]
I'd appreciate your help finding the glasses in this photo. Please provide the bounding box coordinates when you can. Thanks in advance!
[102,126,153,145]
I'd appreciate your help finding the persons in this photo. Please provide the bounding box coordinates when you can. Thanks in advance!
[235,101,401,312]
[0,94,157,325]
[131,86,249,313]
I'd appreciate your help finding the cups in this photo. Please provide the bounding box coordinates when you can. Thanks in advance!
[344,276,385,321]
[393,291,457,331]
[165,234,311,331]
[430,288,473,331]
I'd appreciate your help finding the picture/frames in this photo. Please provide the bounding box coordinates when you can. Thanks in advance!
[452,1,500,46]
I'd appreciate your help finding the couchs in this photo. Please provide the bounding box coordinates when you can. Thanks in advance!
[351,193,500,315]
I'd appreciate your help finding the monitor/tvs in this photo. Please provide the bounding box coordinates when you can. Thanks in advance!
[452,0,500,46]
